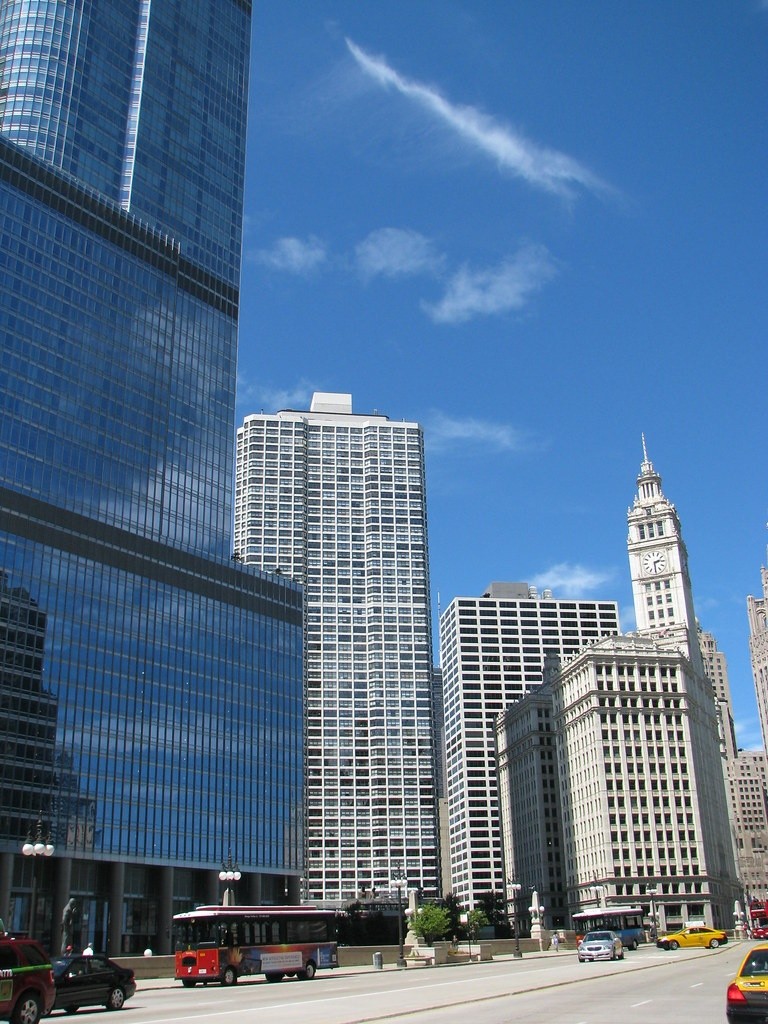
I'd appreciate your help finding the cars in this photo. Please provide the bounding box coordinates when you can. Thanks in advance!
[656,926,728,951]
[577,930,625,962]
[0,930,56,1024]
[726,941,768,1024]
[40,955,136,1018]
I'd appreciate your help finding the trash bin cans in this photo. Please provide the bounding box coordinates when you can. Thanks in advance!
[372,951,383,969]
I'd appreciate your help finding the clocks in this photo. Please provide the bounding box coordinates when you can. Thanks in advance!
[642,551,667,575]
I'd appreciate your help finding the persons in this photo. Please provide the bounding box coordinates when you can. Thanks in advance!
[650,925,657,944]
[83,943,94,955]
[61,898,76,954]
[753,896,758,903]
[553,931,560,952]
[743,921,752,940]
[66,946,76,954]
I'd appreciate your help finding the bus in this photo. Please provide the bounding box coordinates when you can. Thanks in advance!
[748,900,768,940]
[571,906,647,952]
[172,905,340,988]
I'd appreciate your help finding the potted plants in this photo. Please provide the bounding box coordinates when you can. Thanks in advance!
[404,951,434,966]
[410,903,451,965]
[458,908,491,961]
[448,951,470,962]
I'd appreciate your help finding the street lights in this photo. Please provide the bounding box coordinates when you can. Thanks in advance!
[506,873,522,958]
[590,876,604,908]
[646,883,658,943]
[22,810,54,940]
[467,911,473,962]
[218,848,241,906]
[390,861,408,967]
